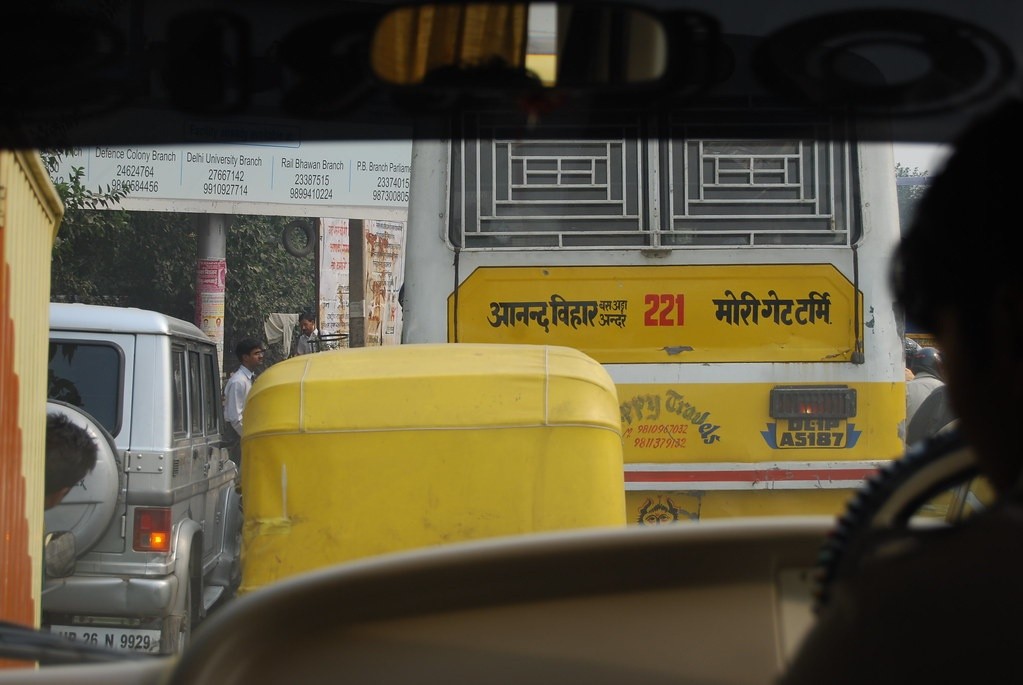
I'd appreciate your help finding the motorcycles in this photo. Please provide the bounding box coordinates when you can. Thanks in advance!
[235,342,627,599]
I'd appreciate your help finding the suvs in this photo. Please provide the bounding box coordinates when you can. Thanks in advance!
[37,297,241,670]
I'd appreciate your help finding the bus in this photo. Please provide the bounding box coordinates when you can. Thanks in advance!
[392,142,913,525]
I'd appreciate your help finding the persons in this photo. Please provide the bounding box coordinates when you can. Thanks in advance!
[223,339,263,464]
[43,412,96,509]
[297,312,319,355]
[203,317,222,331]
[904,337,955,440]
[776,97,1023,685]
